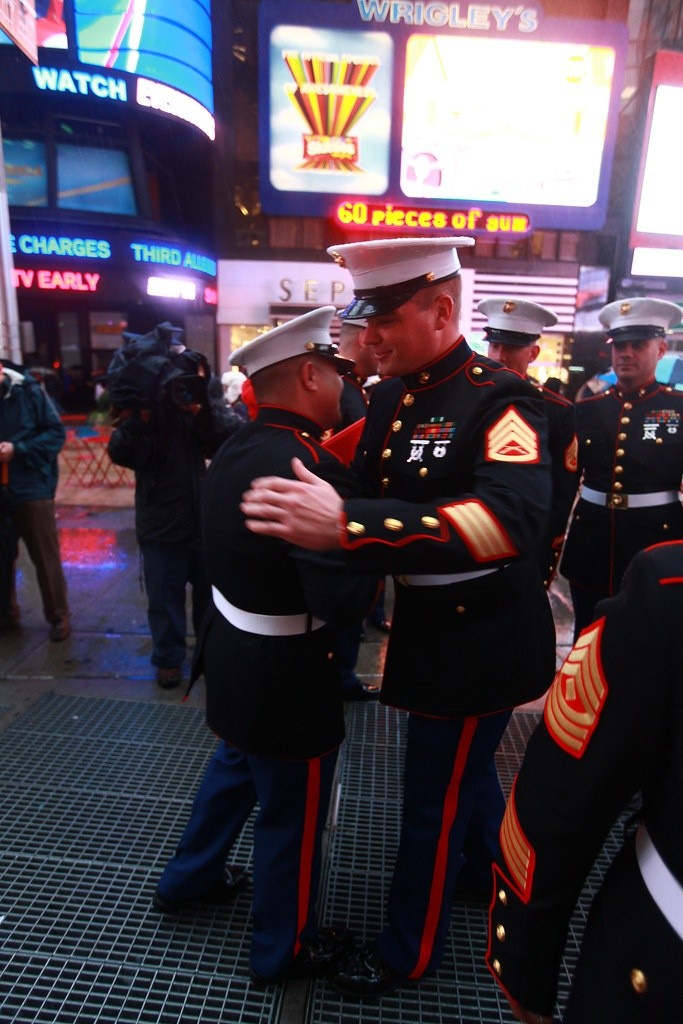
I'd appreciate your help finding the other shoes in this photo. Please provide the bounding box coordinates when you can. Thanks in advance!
[50,617,71,641]
[0,617,20,628]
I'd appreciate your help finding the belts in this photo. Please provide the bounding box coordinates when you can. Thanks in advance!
[579,484,679,510]
[396,564,510,586]
[635,820,683,940]
[211,584,326,636]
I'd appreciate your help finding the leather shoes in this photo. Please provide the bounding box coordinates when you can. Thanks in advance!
[345,684,381,702]
[452,868,492,905]
[153,865,248,911]
[333,940,396,999]
[371,620,391,632]
[251,928,351,984]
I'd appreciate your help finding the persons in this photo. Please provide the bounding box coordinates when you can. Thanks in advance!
[106,344,230,691]
[0,359,72,641]
[153,236,683,1024]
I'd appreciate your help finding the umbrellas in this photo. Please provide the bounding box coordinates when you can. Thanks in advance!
[181,597,218,700]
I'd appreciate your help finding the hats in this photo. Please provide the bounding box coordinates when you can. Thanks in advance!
[598,298,683,344]
[228,305,356,378]
[327,236,475,320]
[336,309,368,327]
[478,297,558,347]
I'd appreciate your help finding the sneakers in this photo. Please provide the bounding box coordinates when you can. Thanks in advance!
[157,668,180,688]
